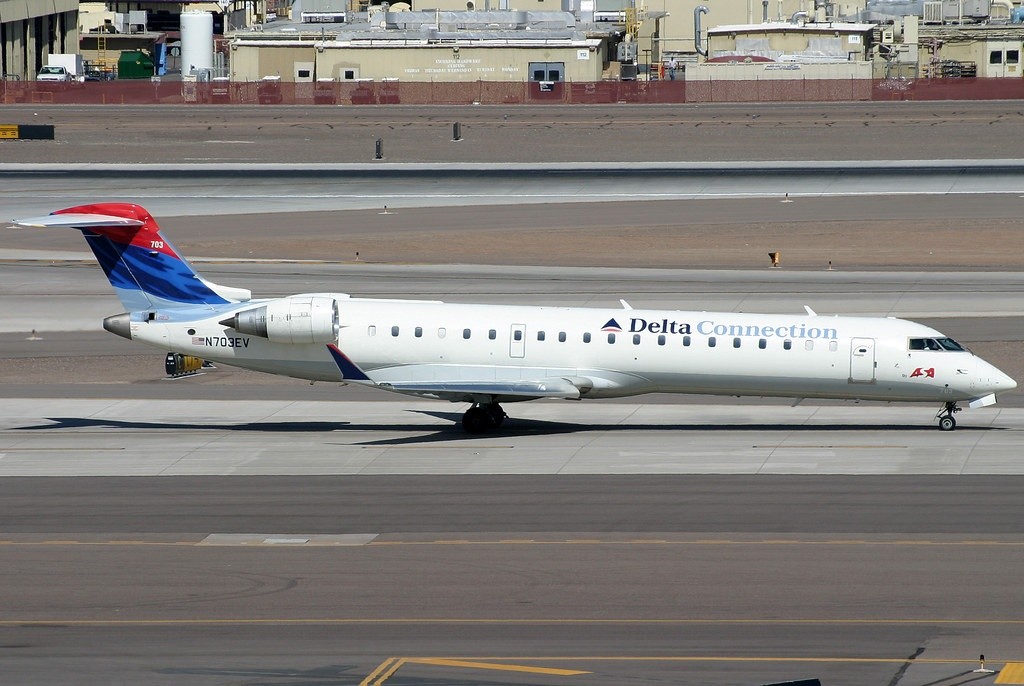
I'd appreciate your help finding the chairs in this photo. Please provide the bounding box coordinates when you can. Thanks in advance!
[45,70,50,73]
[42,69,47,73]
[59,70,64,74]
[912,339,925,349]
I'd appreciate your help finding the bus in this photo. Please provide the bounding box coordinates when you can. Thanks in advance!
[266,11,277,23]
[301,11,346,24]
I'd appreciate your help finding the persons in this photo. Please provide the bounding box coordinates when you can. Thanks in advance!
[667,57,678,80]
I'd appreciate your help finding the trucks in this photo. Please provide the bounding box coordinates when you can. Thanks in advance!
[36,54,85,82]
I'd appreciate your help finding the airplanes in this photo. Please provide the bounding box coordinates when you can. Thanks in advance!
[11,202,1017,436]
